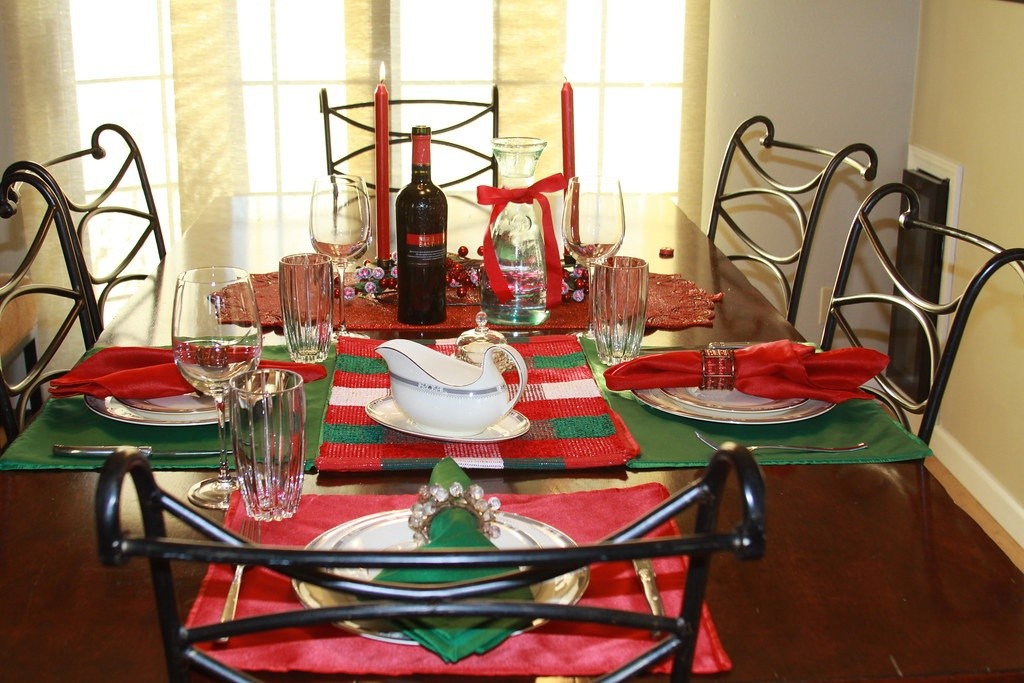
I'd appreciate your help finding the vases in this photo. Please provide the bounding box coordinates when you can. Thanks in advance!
[476,137,552,326]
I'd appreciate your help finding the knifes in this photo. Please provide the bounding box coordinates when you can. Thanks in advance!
[639,343,815,352]
[52,444,232,458]
[633,558,664,616]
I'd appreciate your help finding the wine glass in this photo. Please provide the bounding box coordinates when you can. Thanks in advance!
[309,175,372,344]
[171,266,262,510]
[561,177,626,340]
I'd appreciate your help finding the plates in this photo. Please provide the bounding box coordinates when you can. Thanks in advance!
[292,509,588,646]
[84,394,229,426]
[364,394,530,443]
[659,387,810,414]
[631,388,836,425]
[113,392,215,414]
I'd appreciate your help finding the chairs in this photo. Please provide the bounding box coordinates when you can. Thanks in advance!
[93,445,770,683]
[699,117,1024,604]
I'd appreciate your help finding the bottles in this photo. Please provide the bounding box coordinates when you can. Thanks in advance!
[450,312,515,374]
[396,126,448,325]
[479,136,551,325]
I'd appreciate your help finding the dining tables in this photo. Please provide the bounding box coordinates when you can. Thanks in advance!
[1,195,928,683]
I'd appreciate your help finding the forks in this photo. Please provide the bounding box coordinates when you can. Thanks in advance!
[214,519,261,643]
[182,329,251,344]
[696,430,868,454]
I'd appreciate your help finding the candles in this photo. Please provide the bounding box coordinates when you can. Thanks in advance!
[561,75,578,253]
[373,62,389,257]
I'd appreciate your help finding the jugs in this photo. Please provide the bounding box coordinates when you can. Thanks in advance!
[375,339,528,437]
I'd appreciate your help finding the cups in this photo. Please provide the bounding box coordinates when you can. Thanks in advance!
[229,369,306,521]
[278,253,334,363]
[593,256,649,366]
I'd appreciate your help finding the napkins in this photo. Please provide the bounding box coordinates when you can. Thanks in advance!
[603,337,891,404]
[47,347,328,402]
[181,483,733,675]
[350,456,539,663]
